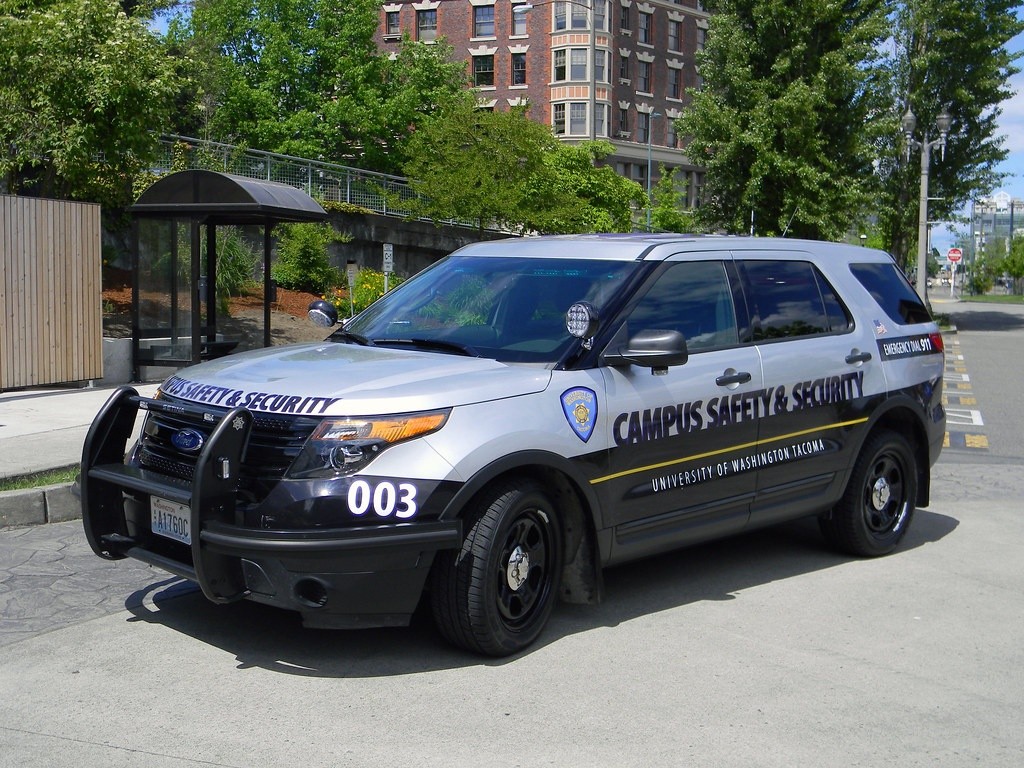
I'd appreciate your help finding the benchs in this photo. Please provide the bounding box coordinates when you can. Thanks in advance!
[151,341,239,368]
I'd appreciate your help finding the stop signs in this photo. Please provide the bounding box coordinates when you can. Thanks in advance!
[948,249,961,261]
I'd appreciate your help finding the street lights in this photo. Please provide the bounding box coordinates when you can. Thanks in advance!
[901,103,953,308]
[646,112,662,231]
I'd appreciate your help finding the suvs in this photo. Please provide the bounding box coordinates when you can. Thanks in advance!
[79,231,945,658]
